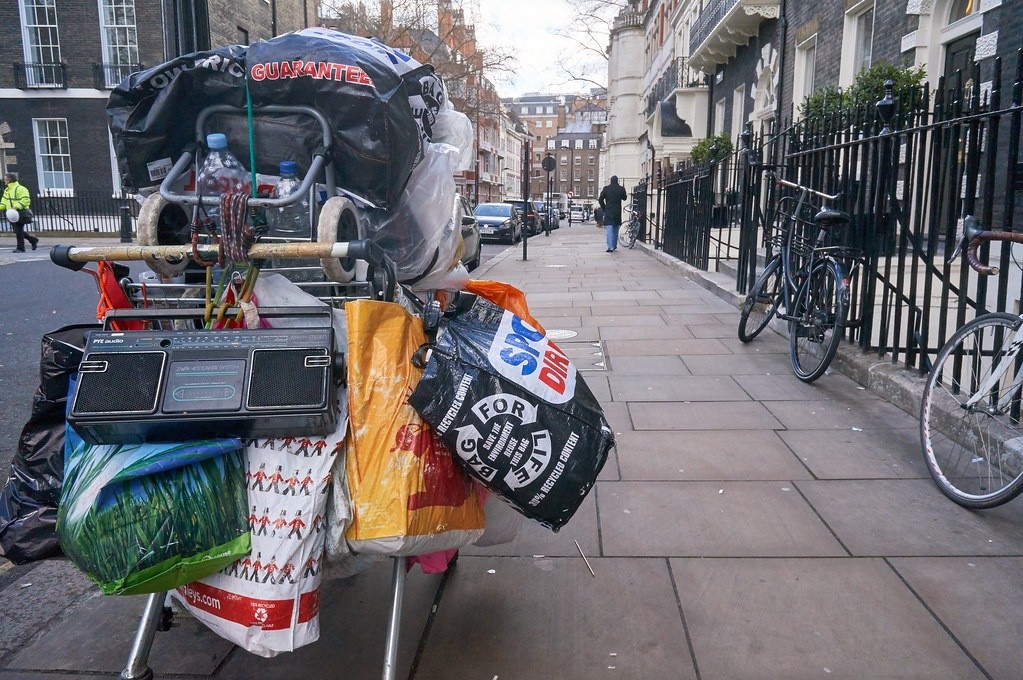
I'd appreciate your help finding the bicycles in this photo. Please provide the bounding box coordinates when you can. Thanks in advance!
[918,214,1023,509]
[618,202,641,249]
[736,169,865,384]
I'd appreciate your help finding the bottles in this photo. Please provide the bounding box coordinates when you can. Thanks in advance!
[195,131,256,295]
[266,162,325,291]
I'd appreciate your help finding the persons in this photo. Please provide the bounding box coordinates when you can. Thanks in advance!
[0,173,39,253]
[599,175,628,252]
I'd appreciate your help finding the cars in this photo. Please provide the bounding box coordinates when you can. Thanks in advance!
[533,200,591,231]
[501,197,543,237]
[473,202,522,245]
[459,195,483,272]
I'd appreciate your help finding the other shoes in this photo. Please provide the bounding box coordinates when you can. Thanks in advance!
[606,250,612,252]
[32,238,39,250]
[12,249,24,253]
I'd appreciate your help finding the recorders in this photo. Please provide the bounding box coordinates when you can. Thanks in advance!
[64,306,345,439]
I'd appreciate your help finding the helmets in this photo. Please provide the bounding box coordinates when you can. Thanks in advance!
[6,209,19,223]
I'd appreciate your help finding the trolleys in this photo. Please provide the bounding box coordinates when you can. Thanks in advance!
[48,238,429,680]
[135,102,366,285]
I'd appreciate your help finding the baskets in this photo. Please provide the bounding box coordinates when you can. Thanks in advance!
[767,196,821,257]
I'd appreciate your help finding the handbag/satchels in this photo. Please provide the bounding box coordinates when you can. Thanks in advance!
[343,299,487,555]
[18,209,33,223]
[1,322,105,565]
[166,369,352,658]
[56,373,252,596]
[399,282,616,534]
